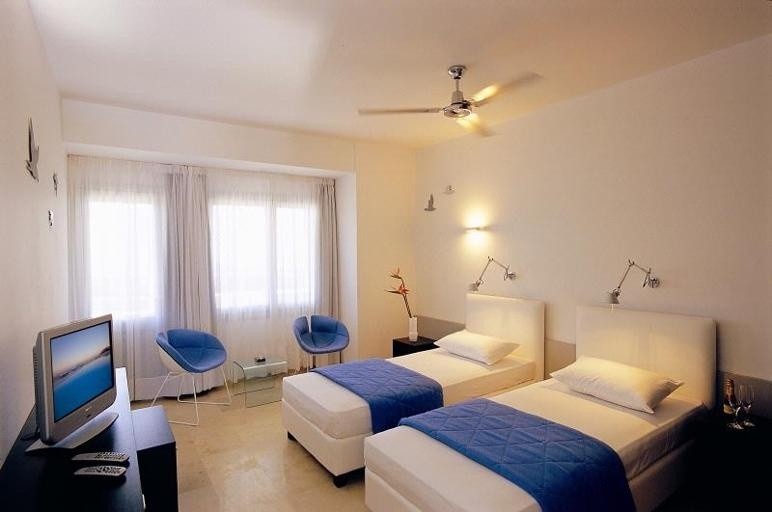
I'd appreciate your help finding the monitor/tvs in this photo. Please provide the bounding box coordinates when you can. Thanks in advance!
[24,314,119,453]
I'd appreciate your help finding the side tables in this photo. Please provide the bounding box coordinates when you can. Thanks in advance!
[232,354,288,407]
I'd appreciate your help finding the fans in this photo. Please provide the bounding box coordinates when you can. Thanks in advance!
[358,64,543,139]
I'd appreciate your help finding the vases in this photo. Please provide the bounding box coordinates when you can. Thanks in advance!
[408,317,418,341]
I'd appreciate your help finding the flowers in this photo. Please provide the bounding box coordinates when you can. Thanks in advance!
[384,268,413,318]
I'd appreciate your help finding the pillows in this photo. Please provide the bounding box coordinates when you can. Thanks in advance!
[435,329,520,365]
[549,357,682,414]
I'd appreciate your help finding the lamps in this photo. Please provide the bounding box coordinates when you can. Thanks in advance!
[605,260,661,305]
[467,255,517,294]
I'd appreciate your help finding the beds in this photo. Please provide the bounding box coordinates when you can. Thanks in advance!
[281,292,546,487]
[363,304,717,512]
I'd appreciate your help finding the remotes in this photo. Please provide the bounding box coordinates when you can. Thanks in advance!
[73,465,126,477]
[72,452,130,463]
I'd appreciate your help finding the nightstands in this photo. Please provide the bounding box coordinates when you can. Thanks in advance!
[393,336,434,356]
[696,403,771,510]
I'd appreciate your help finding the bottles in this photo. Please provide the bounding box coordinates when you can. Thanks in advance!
[723,378,738,423]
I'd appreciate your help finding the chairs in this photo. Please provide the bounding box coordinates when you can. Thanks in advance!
[150,329,232,427]
[293,315,350,372]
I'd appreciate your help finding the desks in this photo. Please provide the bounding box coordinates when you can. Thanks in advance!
[1,368,178,512]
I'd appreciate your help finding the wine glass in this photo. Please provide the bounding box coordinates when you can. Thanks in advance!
[739,383,754,427]
[728,386,744,430]
